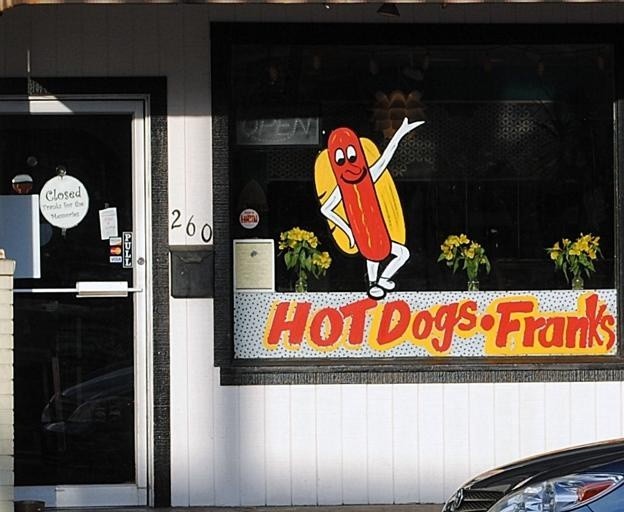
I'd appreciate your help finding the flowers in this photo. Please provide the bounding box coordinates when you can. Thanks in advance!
[274,225,333,292]
[547,233,605,289]
[434,233,491,291]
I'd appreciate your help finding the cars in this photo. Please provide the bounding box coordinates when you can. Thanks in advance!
[30,366,133,482]
[442,437,624,512]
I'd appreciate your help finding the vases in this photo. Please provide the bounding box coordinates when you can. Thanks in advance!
[467,280,479,291]
[294,277,309,292]
[571,270,583,289]
